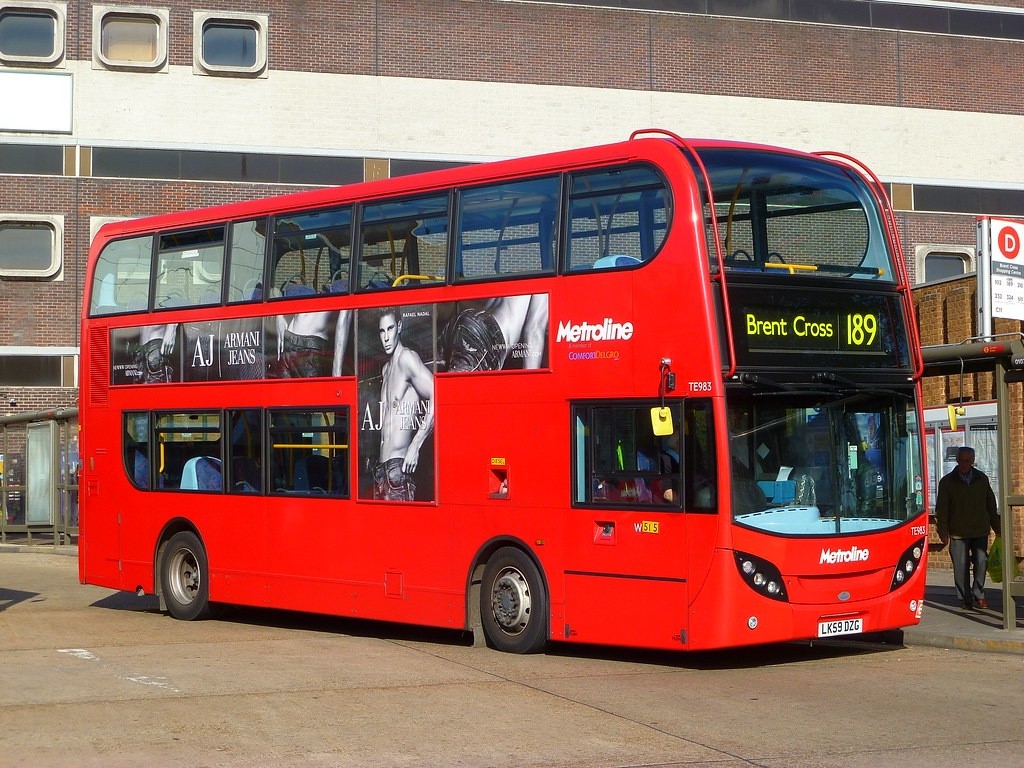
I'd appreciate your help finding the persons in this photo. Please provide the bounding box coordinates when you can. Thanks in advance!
[131,321,180,385]
[356,302,435,503]
[934,446,1001,610]
[571,399,754,514]
[262,309,353,378]
[242,432,287,493]
[434,293,549,374]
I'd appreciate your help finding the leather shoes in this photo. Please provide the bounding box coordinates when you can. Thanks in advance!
[973,595,989,608]
[964,605,972,610]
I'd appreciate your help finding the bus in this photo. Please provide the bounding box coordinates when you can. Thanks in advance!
[77,126,966,658]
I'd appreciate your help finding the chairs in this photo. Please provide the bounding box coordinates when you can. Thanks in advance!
[633,442,660,503]
[125,429,349,499]
[94,264,395,315]
[771,481,796,504]
[758,480,775,498]
